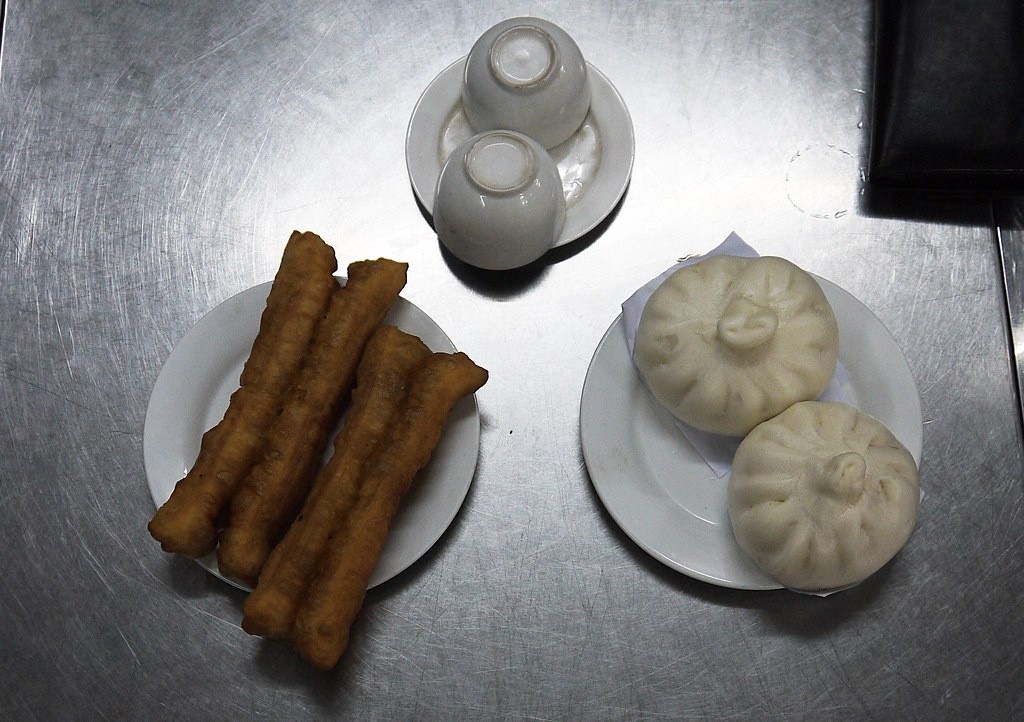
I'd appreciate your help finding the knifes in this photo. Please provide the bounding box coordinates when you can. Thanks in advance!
[993,196,1024,430]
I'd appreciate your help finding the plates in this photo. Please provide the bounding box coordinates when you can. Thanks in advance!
[142,275,480,594]
[579,271,922,590]
[407,54,634,249]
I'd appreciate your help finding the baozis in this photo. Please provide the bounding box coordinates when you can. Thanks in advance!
[633,256,842,435]
[730,400,917,591]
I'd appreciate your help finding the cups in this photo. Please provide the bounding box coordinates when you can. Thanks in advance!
[435,130,566,270]
[463,16,590,151]
